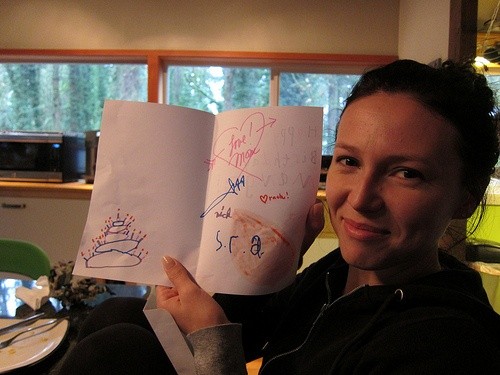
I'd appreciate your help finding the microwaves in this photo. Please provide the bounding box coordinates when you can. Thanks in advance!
[1,130,86,183]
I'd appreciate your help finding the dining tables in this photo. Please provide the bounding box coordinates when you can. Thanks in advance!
[1,277,88,375]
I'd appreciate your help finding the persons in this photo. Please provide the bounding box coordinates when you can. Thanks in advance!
[52,59,500,375]
